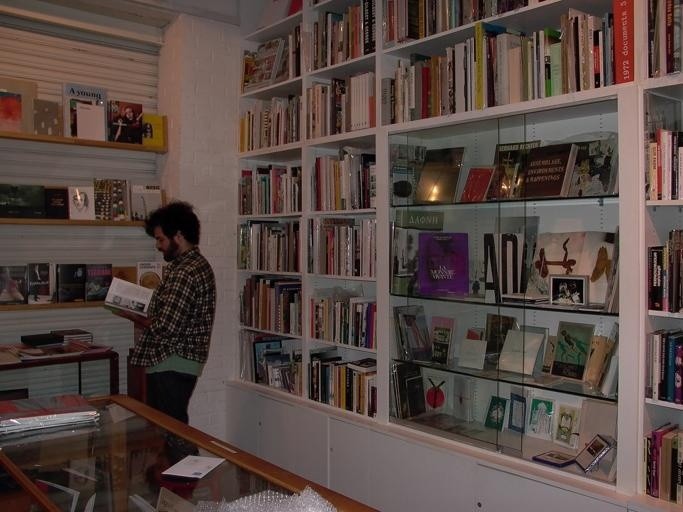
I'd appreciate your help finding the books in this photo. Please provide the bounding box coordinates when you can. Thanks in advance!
[1,75,166,147]
[381,2,680,507]
[236,1,377,416]
[2,180,166,367]
[0,390,99,434]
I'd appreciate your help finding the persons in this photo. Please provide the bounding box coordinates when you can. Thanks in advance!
[112,203,216,483]
